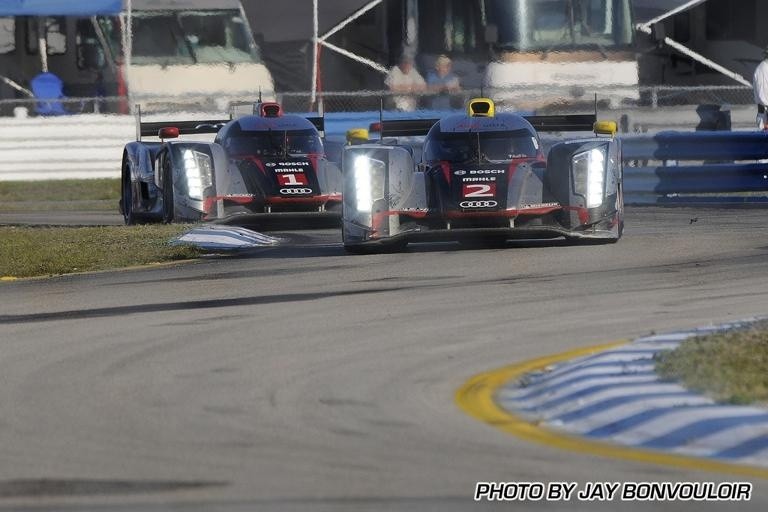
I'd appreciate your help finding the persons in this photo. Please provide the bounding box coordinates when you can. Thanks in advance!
[425,55,461,110]
[752,49,768,132]
[384,53,427,112]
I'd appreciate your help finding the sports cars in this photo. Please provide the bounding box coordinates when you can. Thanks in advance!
[341,77,625,254]
[118,85,346,226]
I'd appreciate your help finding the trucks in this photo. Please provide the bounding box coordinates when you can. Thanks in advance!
[1,1,277,118]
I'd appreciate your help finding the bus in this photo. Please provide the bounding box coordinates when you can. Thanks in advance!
[239,1,666,119]
